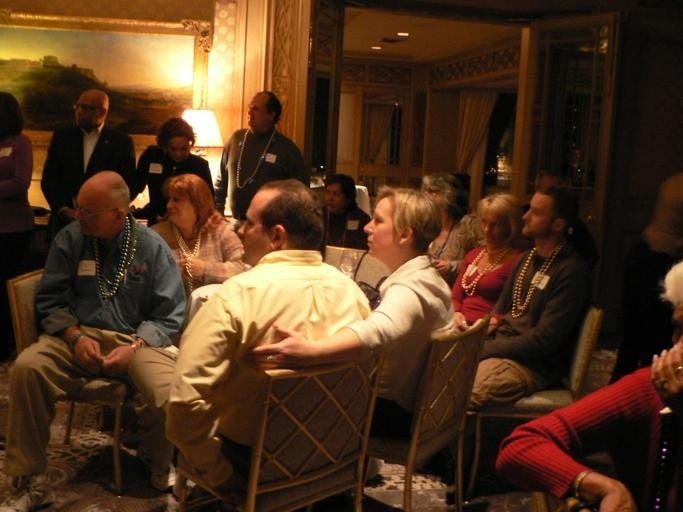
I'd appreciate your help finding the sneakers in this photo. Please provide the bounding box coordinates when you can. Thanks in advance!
[0,471,55,512]
[149,461,178,491]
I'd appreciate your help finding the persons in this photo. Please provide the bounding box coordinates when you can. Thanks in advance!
[0,170,186,511]
[164,178,370,512]
[220,91,308,221]
[149,172,251,295]
[41,88,136,243]
[0,92,32,362]
[317,171,681,511]
[245,184,455,441]
[130,117,214,227]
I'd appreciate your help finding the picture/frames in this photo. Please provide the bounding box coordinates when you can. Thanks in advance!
[0,8,213,167]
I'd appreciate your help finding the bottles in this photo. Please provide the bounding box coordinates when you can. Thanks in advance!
[571,128,584,186]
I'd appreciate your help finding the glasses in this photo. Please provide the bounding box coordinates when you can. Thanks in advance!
[72,195,118,217]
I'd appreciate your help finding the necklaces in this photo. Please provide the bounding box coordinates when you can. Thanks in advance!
[236,128,276,188]
[92,216,138,299]
[170,223,202,294]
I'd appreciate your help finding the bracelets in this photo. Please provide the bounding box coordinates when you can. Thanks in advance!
[130,339,143,352]
[67,333,88,352]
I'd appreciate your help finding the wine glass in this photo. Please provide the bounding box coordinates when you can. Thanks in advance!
[339,249,359,279]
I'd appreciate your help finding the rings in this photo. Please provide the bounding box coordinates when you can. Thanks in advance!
[189,258,191,264]
[266,354,276,363]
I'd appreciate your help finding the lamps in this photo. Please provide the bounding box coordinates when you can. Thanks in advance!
[180,109,224,158]
